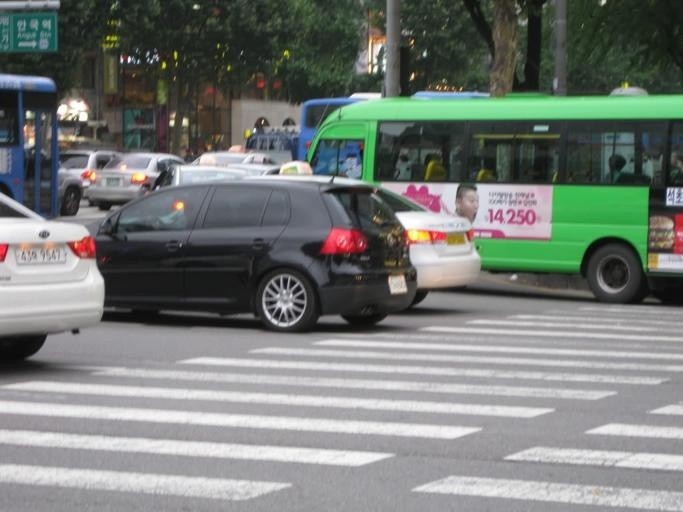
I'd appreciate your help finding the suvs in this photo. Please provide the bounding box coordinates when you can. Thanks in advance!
[83,177,415,335]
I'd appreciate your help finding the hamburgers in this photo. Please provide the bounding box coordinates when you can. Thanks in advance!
[648,215,676,253]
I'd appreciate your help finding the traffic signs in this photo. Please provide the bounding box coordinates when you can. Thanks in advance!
[0,12,58,53]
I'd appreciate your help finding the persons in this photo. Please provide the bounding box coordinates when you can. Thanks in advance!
[450,182,479,228]
[669,149,682,182]
[604,152,625,184]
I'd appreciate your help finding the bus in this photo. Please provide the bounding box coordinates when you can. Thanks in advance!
[306,84,682,311]
[0,72,58,219]
[297,90,489,178]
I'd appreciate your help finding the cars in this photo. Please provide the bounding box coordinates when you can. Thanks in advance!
[0,193,105,361]
[246,170,481,309]
[25,122,299,217]
